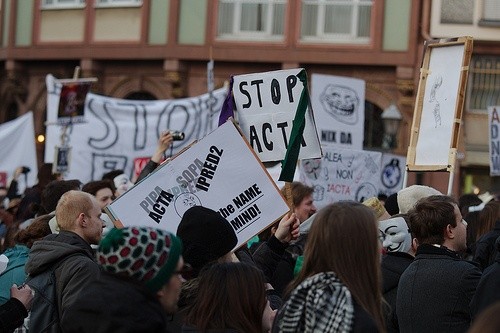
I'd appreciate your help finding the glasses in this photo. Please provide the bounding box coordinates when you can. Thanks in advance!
[173,263,192,281]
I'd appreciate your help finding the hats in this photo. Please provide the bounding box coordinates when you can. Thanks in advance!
[397,184,444,214]
[96,225,182,290]
[176,205,238,267]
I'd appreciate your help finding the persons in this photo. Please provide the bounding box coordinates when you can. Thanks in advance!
[0,132,500,333]
[271,200,389,333]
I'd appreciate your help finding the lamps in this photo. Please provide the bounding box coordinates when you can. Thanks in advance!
[380,102,402,150]
